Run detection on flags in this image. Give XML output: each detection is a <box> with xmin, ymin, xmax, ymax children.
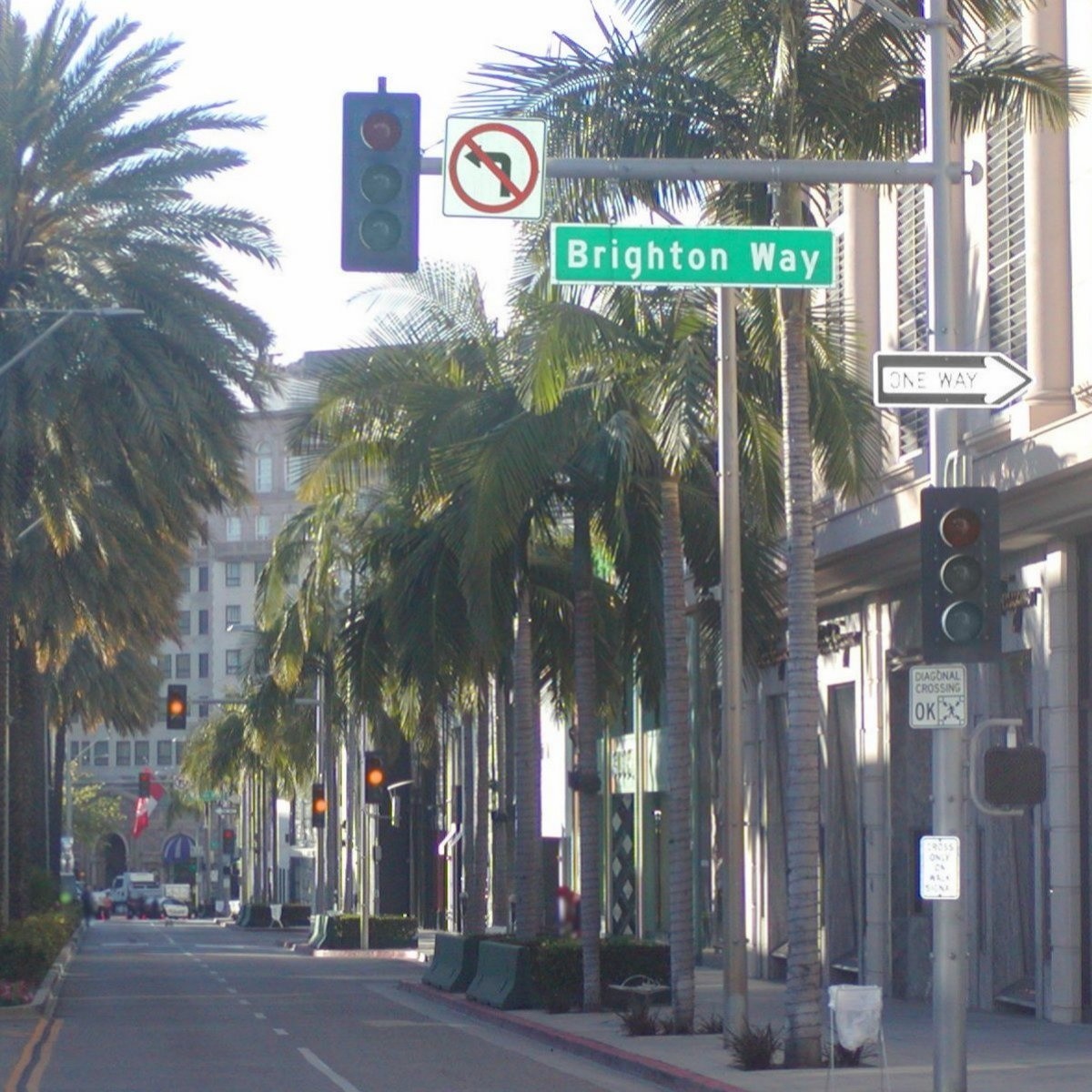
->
<box><xmin>131</xmin><ymin>764</ymin><xmax>166</xmax><ymax>838</ymax></box>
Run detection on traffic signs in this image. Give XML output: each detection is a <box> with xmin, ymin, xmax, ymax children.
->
<box><xmin>873</xmin><ymin>346</ymin><xmax>1033</xmax><ymax>415</ymax></box>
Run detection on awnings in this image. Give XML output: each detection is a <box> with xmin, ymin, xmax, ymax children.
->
<box><xmin>162</xmin><ymin>834</ymin><xmax>197</xmax><ymax>858</ymax></box>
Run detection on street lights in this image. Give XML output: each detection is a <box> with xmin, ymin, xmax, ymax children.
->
<box><xmin>224</xmin><ymin>623</ymin><xmax>339</xmax><ymax>923</ymax></box>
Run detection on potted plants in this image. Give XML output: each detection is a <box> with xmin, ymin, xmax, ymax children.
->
<box><xmin>236</xmin><ymin>901</ymin><xmax>673</xmax><ymax>1010</ymax></box>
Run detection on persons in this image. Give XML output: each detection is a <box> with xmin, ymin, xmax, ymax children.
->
<box><xmin>554</xmin><ymin>885</ymin><xmax>602</xmax><ymax>941</ymax></box>
<box><xmin>81</xmin><ymin>883</ymin><xmax>114</xmax><ymax>928</ymax></box>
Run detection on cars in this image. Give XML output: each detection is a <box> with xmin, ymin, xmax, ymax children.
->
<box><xmin>89</xmin><ymin>872</ymin><xmax>198</xmax><ymax>920</ymax></box>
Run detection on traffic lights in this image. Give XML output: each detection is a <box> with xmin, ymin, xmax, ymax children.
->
<box><xmin>166</xmin><ymin>686</ymin><xmax>188</xmax><ymax>731</ymax></box>
<box><xmin>979</xmin><ymin>744</ymin><xmax>1051</xmax><ymax>811</ymax></box>
<box><xmin>223</xmin><ymin>824</ymin><xmax>234</xmax><ymax>855</ymax></box>
<box><xmin>139</xmin><ymin>773</ymin><xmax>149</xmax><ymax>798</ymax></box>
<box><xmin>342</xmin><ymin>85</ymin><xmax>419</xmax><ymax>279</ymax></box>
<box><xmin>359</xmin><ymin>751</ymin><xmax>389</xmax><ymax>805</ymax></box>
<box><xmin>917</xmin><ymin>479</ymin><xmax>1006</xmax><ymax>670</ymax></box>
<box><xmin>311</xmin><ymin>782</ymin><xmax>325</xmax><ymax>828</ymax></box>
